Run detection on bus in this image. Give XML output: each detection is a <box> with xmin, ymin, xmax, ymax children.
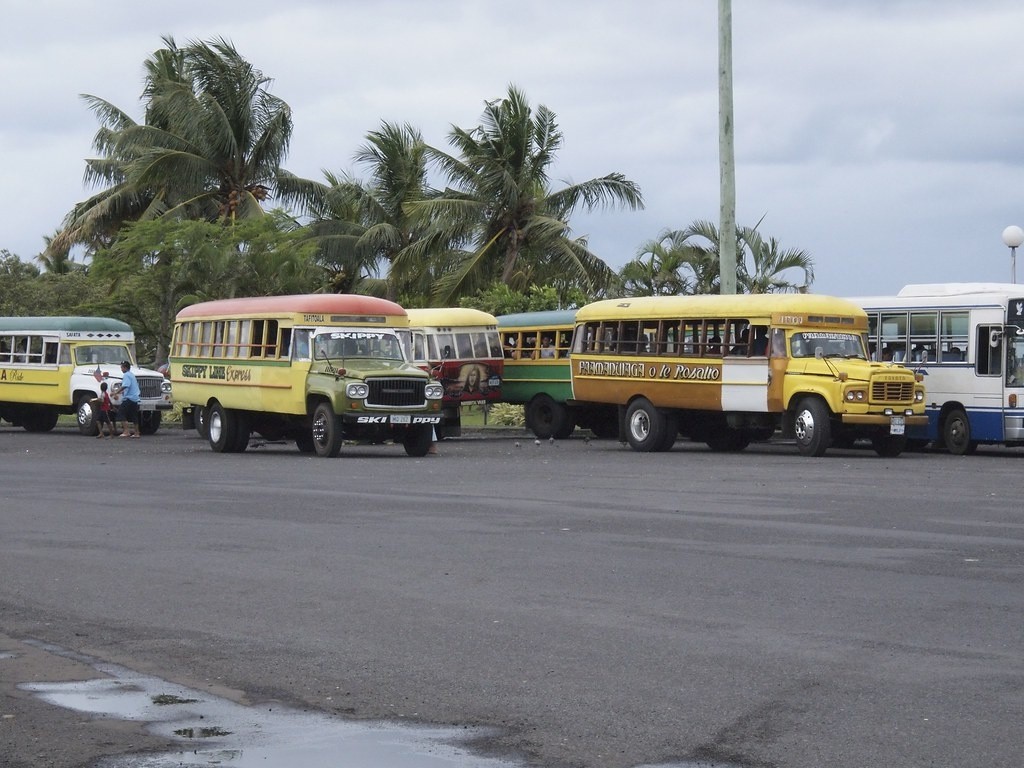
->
<box><xmin>569</xmin><ymin>293</ymin><xmax>929</xmax><ymax>459</ymax></box>
<box><xmin>494</xmin><ymin>308</ymin><xmax>620</xmax><ymax>440</ymax></box>
<box><xmin>0</xmin><ymin>316</ymin><xmax>173</xmax><ymax>435</ymax></box>
<box><xmin>402</xmin><ymin>307</ymin><xmax>504</xmax><ymax>440</ymax></box>
<box><xmin>841</xmin><ymin>280</ymin><xmax>1023</xmax><ymax>457</ymax></box>
<box><xmin>166</xmin><ymin>294</ymin><xmax>448</xmax><ymax>458</ymax></box>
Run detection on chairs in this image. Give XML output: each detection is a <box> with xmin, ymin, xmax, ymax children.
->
<box><xmin>102</xmin><ymin>348</ymin><xmax>117</xmax><ymax>361</ymax></box>
<box><xmin>927</xmin><ymin>355</ymin><xmax>936</xmax><ymax>361</ymax></box>
<box><xmin>332</xmin><ymin>339</ymin><xmax>358</xmax><ymax>355</ymax></box>
<box><xmin>942</xmin><ymin>354</ymin><xmax>960</xmax><ymax>361</ymax></box>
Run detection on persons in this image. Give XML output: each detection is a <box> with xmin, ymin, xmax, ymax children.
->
<box><xmin>707</xmin><ymin>336</ymin><xmax>721</xmax><ymax>354</ymax></box>
<box><xmin>332</xmin><ymin>338</ymin><xmax>358</xmax><ymax>356</ymax></box>
<box><xmin>870</xmin><ymin>341</ymin><xmax>961</xmax><ymax>362</ymax></box>
<box><xmin>110</xmin><ymin>361</ymin><xmax>141</xmax><ymax>438</ymax></box>
<box><xmin>731</xmin><ymin>326</ymin><xmax>769</xmax><ymax>356</ymax></box>
<box><xmin>610</xmin><ymin>327</ymin><xmax>648</xmax><ymax>351</ymax></box>
<box><xmin>96</xmin><ymin>382</ymin><xmax>113</xmax><ymax>439</ymax></box>
<box><xmin>1013</xmin><ymin>355</ymin><xmax>1024</xmax><ymax>386</ymax></box>
<box><xmin>504</xmin><ymin>333</ymin><xmax>567</xmax><ymax>359</ymax></box>
<box><xmin>806</xmin><ymin>338</ymin><xmax>834</xmax><ymax>355</ymax></box>
<box><xmin>0</xmin><ymin>338</ymin><xmax>34</xmax><ymax>362</ymax></box>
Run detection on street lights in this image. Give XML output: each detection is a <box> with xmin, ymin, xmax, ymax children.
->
<box><xmin>1001</xmin><ymin>223</ymin><xmax>1024</xmax><ymax>282</ymax></box>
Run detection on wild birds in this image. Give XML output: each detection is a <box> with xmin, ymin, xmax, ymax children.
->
<box><xmin>535</xmin><ymin>435</ymin><xmax>541</xmax><ymax>446</ymax></box>
<box><xmin>515</xmin><ymin>442</ymin><xmax>519</xmax><ymax>448</ymax></box>
<box><xmin>549</xmin><ymin>435</ymin><xmax>555</xmax><ymax>445</ymax></box>
<box><xmin>584</xmin><ymin>435</ymin><xmax>594</xmax><ymax>446</ymax></box>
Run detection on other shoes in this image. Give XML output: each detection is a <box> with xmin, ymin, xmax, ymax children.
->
<box><xmin>130</xmin><ymin>435</ymin><xmax>140</xmax><ymax>437</ymax></box>
<box><xmin>105</xmin><ymin>436</ymin><xmax>113</xmax><ymax>440</ymax></box>
<box><xmin>120</xmin><ymin>433</ymin><xmax>131</xmax><ymax>437</ymax></box>
<box><xmin>97</xmin><ymin>433</ymin><xmax>104</xmax><ymax>438</ymax></box>
